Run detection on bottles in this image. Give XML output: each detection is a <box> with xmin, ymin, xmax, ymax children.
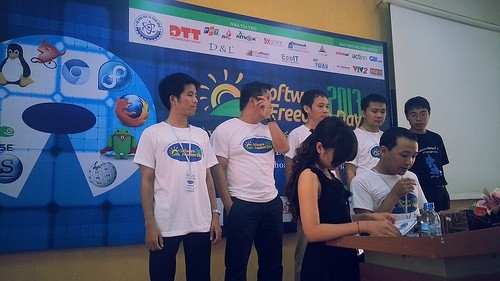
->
<box><xmin>420</xmin><ymin>203</ymin><xmax>442</xmax><ymax>237</ymax></box>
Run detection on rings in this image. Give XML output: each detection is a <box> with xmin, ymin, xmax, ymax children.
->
<box><xmin>357</xmin><ymin>221</ymin><xmax>362</xmax><ymax>234</ymax></box>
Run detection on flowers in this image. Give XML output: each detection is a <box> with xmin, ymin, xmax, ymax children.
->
<box><xmin>468</xmin><ymin>183</ymin><xmax>500</xmax><ymax>216</ymax></box>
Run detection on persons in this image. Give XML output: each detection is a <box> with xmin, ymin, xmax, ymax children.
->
<box><xmin>350</xmin><ymin>127</ymin><xmax>428</xmax><ymax>220</ymax></box>
<box><xmin>288</xmin><ymin>116</ymin><xmax>399</xmax><ymax>281</ymax></box>
<box><xmin>343</xmin><ymin>93</ymin><xmax>388</xmax><ymax>189</ymax></box>
<box><xmin>211</xmin><ymin>81</ymin><xmax>290</xmax><ymax>281</ymax></box>
<box><xmin>132</xmin><ymin>71</ymin><xmax>223</xmax><ymax>281</ymax></box>
<box><xmin>405</xmin><ymin>95</ymin><xmax>451</xmax><ymax>213</ymax></box>
<box><xmin>283</xmin><ymin>88</ymin><xmax>345</xmax><ymax>281</ymax></box>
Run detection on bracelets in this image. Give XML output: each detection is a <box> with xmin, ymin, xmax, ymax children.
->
<box><xmin>213</xmin><ymin>208</ymin><xmax>223</xmax><ymax>218</ymax></box>
<box><xmin>265</xmin><ymin>116</ymin><xmax>276</xmax><ymax>123</ymax></box>
